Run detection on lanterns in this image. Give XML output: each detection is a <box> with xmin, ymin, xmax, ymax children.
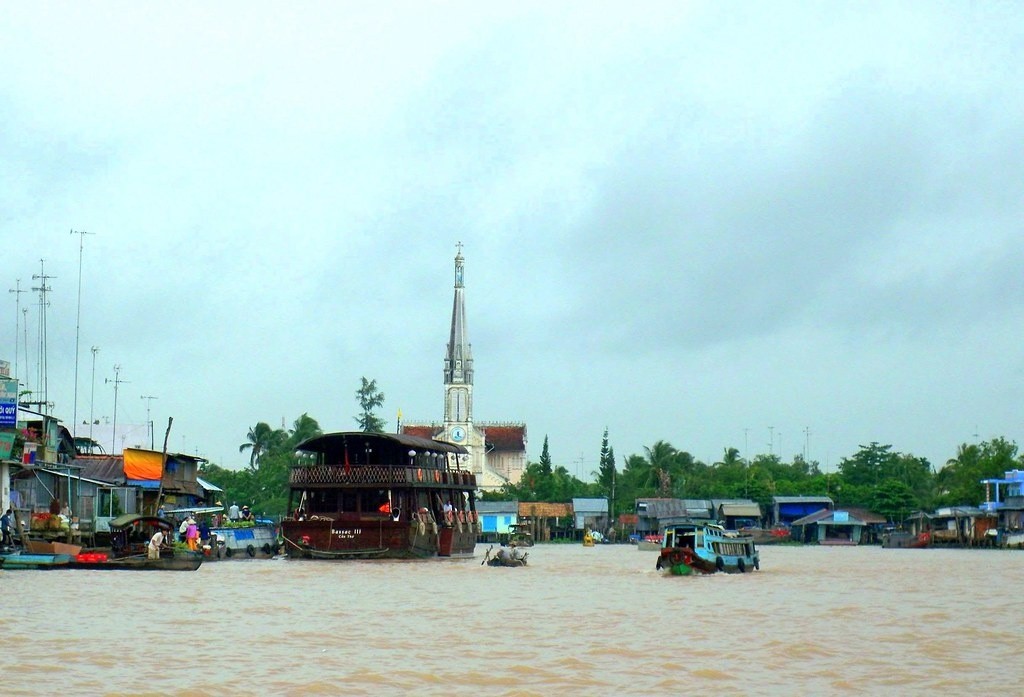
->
<box><xmin>409</xmin><ymin>450</ymin><xmax>469</xmax><ymax>464</ymax></box>
<box><xmin>295</xmin><ymin>449</ymin><xmax>315</xmax><ymax>465</ymax></box>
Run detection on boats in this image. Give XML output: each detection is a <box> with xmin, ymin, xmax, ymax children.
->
<box><xmin>282</xmin><ymin>430</ymin><xmax>481</xmax><ymax>561</ymax></box>
<box><xmin>656</xmin><ymin>523</ymin><xmax>760</xmax><ymax>575</ymax></box>
<box><xmin>636</xmin><ymin>534</ymin><xmax>664</xmax><ymax>551</ymax></box>
<box><xmin>583</xmin><ymin>533</ymin><xmax>594</xmax><ymax>547</ymax></box>
<box><xmin>487</xmin><ymin>552</ymin><xmax>528</xmax><ymax>566</ymax></box>
<box><xmin>0</xmin><ymin>512</ymin><xmax>206</xmax><ymax>571</ymax></box>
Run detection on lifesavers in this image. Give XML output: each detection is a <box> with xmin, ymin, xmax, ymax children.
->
<box><xmin>225</xmin><ymin>547</ymin><xmax>232</xmax><ymax>559</ymax></box>
<box><xmin>247</xmin><ymin>544</ymin><xmax>256</xmax><ymax>557</ymax></box>
<box><xmin>273</xmin><ymin>544</ymin><xmax>279</xmax><ymax>555</ymax></box>
<box><xmin>716</xmin><ymin>555</ymin><xmax>726</xmax><ymax>570</ymax></box>
<box><xmin>669</xmin><ymin>550</ymin><xmax>686</xmax><ymax>566</ymax></box>
<box><xmin>754</xmin><ymin>558</ymin><xmax>759</xmax><ymax>570</ymax></box>
<box><xmin>738</xmin><ymin>557</ymin><xmax>746</xmax><ymax>572</ymax></box>
<box><xmin>435</xmin><ymin>469</ymin><xmax>440</xmax><ymax>483</ymax></box>
<box><xmin>417</xmin><ymin>468</ymin><xmax>424</xmax><ymax>481</ymax></box>
<box><xmin>459</xmin><ymin>510</ymin><xmax>464</xmax><ymax>523</ymax></box>
<box><xmin>264</xmin><ymin>543</ymin><xmax>272</xmax><ymax>555</ymax></box>
<box><xmin>448</xmin><ymin>511</ymin><xmax>453</xmax><ymax>521</ymax></box>
<box><xmin>467</xmin><ymin>511</ymin><xmax>474</xmax><ymax>522</ymax></box>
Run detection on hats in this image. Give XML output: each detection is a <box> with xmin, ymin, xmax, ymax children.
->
<box><xmin>212</xmin><ymin>514</ymin><xmax>216</xmax><ymax>517</ymax></box>
<box><xmin>222</xmin><ymin>514</ymin><xmax>227</xmax><ymax>519</ymax></box>
<box><xmin>242</xmin><ymin>506</ymin><xmax>249</xmax><ymax>509</ymax></box>
<box><xmin>188</xmin><ymin>519</ymin><xmax>196</xmax><ymax>524</ymax></box>
<box><xmin>159</xmin><ymin>504</ymin><xmax>165</xmax><ymax>508</ymax></box>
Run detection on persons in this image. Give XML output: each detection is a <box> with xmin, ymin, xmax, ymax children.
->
<box><xmin>148</xmin><ymin>527</ymin><xmax>175</xmax><ymax>558</ymax></box>
<box><xmin>497</xmin><ymin>541</ymin><xmax>521</xmax><ymax>560</ymax></box>
<box><xmin>50</xmin><ymin>499</ymin><xmax>72</xmax><ymax>519</ymax></box>
<box><xmin>444</xmin><ymin>500</ymin><xmax>452</xmax><ymax>522</ymax></box>
<box><xmin>0</xmin><ymin>508</ymin><xmax>12</xmax><ymax>543</ymax></box>
<box><xmin>178</xmin><ymin>516</ymin><xmax>211</xmax><ymax>551</ymax></box>
<box><xmin>675</xmin><ymin>537</ymin><xmax>679</xmax><ymax>548</ymax></box>
<box><xmin>158</xmin><ymin>501</ymin><xmax>254</xmax><ymax>529</ymax></box>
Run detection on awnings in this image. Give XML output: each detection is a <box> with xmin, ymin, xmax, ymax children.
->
<box><xmin>197</xmin><ymin>477</ymin><xmax>225</xmax><ymax>492</ymax></box>
<box><xmin>473</xmin><ymin>514</ymin><xmax>517</xmax><ymax>534</ymax></box>
